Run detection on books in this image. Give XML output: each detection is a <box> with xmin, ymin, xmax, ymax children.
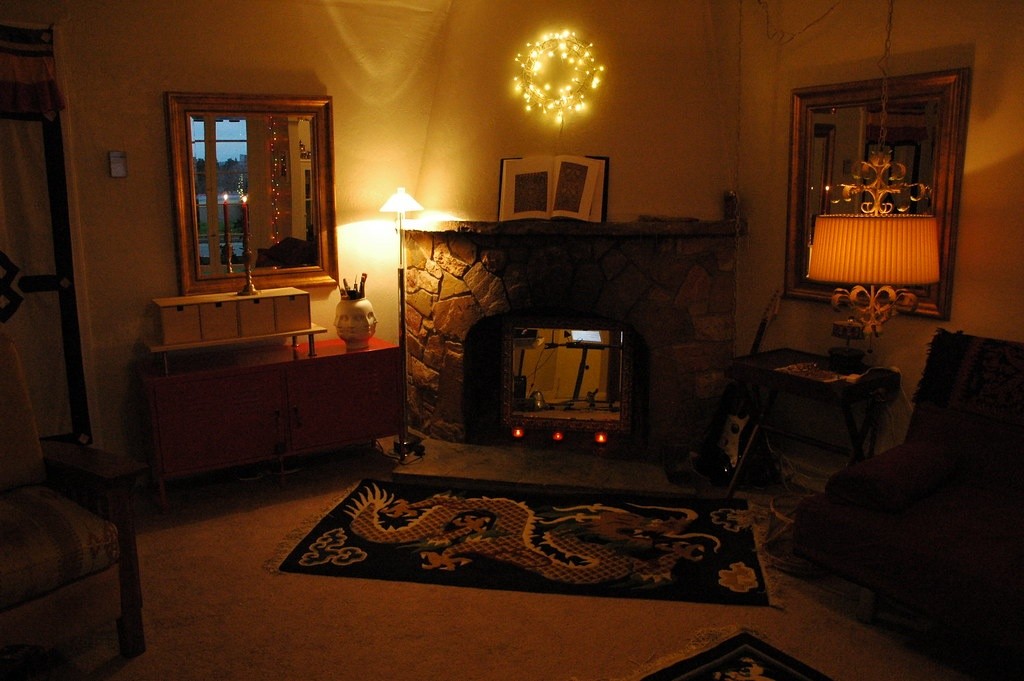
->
<box><xmin>496</xmin><ymin>154</ymin><xmax>611</xmax><ymax>226</ymax></box>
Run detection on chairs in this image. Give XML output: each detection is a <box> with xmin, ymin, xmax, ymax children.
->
<box><xmin>0</xmin><ymin>334</ymin><xmax>147</xmax><ymax>658</ymax></box>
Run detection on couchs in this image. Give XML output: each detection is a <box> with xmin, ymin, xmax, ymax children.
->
<box><xmin>793</xmin><ymin>328</ymin><xmax>1024</xmax><ymax>681</ymax></box>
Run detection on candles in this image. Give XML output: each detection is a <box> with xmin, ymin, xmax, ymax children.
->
<box><xmin>242</xmin><ymin>204</ymin><xmax>248</xmax><ymax>235</ymax></box>
<box><xmin>223</xmin><ymin>202</ymin><xmax>231</xmax><ymax>233</ymax></box>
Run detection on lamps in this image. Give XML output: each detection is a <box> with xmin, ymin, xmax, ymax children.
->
<box><xmin>808</xmin><ymin>0</ymin><xmax>944</xmax><ymax>337</ymax></box>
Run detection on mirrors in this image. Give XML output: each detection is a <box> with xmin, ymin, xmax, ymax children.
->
<box><xmin>782</xmin><ymin>66</ymin><xmax>971</xmax><ymax>320</ymax></box>
<box><xmin>166</xmin><ymin>91</ymin><xmax>340</xmax><ymax>296</ymax></box>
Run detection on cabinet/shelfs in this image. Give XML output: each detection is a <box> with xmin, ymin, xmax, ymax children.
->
<box><xmin>141</xmin><ymin>336</ymin><xmax>406</xmax><ymax>506</ymax></box>
<box><xmin>144</xmin><ymin>286</ymin><xmax>327</xmax><ymax>374</ymax></box>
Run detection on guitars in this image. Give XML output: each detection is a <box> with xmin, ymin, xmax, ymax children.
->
<box><xmin>696</xmin><ymin>283</ymin><xmax>786</xmax><ymax>487</ymax></box>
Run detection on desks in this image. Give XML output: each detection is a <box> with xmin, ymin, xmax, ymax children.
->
<box><xmin>730</xmin><ymin>347</ymin><xmax>900</xmax><ymax>466</ymax></box>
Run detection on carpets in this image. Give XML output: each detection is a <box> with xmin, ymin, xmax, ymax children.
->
<box><xmin>264</xmin><ymin>477</ymin><xmax>786</xmax><ymax>610</ymax></box>
<box><xmin>616</xmin><ymin>624</ymin><xmax>837</xmax><ymax>681</ymax></box>
